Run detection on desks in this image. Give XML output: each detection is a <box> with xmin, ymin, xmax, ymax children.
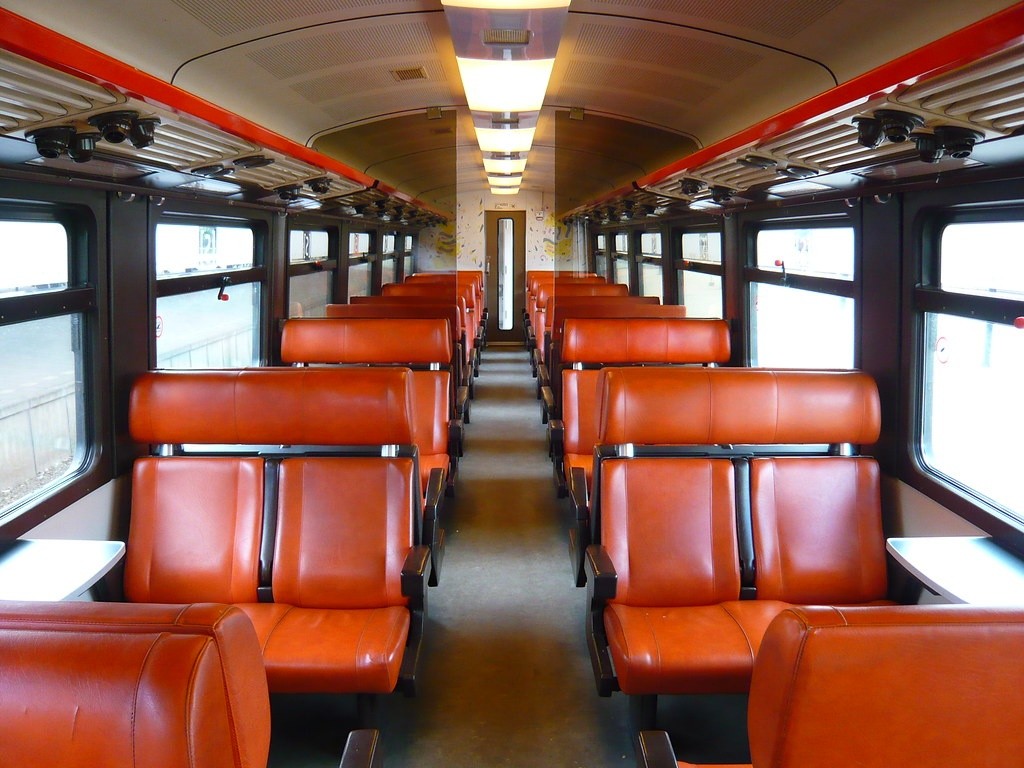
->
<box><xmin>0</xmin><ymin>538</ymin><xmax>127</xmax><ymax>604</ymax></box>
<box><xmin>886</xmin><ymin>534</ymin><xmax>1024</xmax><ymax>609</ymax></box>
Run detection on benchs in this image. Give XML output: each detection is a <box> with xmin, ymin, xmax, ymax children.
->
<box><xmin>524</xmin><ymin>267</ymin><xmax>1024</xmax><ymax>768</ymax></box>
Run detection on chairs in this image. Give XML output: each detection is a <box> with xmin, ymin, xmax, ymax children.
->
<box><xmin>0</xmin><ymin>270</ymin><xmax>488</xmax><ymax>767</ymax></box>
